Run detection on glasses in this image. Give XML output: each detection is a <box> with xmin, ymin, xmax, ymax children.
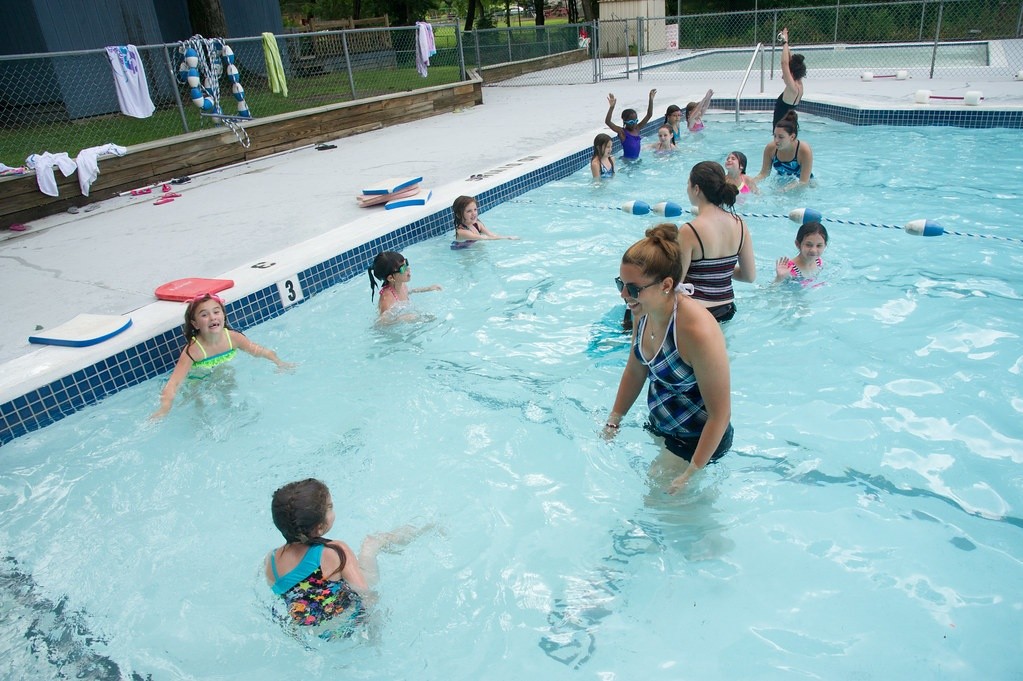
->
<box><xmin>624</xmin><ymin>119</ymin><xmax>638</xmax><ymax>125</ymax></box>
<box><xmin>392</xmin><ymin>258</ymin><xmax>409</xmax><ymax>275</ymax></box>
<box><xmin>614</xmin><ymin>275</ymin><xmax>663</xmax><ymax>298</ymax></box>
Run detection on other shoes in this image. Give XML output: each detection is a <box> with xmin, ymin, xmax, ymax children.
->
<box><xmin>83</xmin><ymin>201</ymin><xmax>101</xmax><ymax>212</ymax></box>
<box><xmin>132</xmin><ymin>188</ymin><xmax>151</xmax><ymax>195</ymax></box>
<box><xmin>67</xmin><ymin>206</ymin><xmax>79</xmax><ymax>215</ymax></box>
<box><xmin>162</xmin><ymin>184</ymin><xmax>170</xmax><ymax>192</ymax></box>
<box><xmin>171</xmin><ymin>176</ymin><xmax>192</xmax><ymax>185</ymax></box>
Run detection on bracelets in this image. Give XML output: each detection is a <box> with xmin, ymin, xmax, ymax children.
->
<box><xmin>606</xmin><ymin>422</ymin><xmax>621</xmax><ymax>429</ymax></box>
<box><xmin>782</xmin><ymin>40</ymin><xmax>788</xmax><ymax>45</ymax></box>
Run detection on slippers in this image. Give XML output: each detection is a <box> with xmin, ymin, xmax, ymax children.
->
<box><xmin>161</xmin><ymin>191</ymin><xmax>182</xmax><ymax>197</ymax></box>
<box><xmin>9</xmin><ymin>223</ymin><xmax>26</xmax><ymax>231</ymax></box>
<box><xmin>153</xmin><ymin>197</ymin><xmax>174</xmax><ymax>206</ymax></box>
<box><xmin>314</xmin><ymin>143</ymin><xmax>336</xmax><ymax>149</ymax></box>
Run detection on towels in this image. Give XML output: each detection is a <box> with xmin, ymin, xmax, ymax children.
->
<box><xmin>25</xmin><ymin>151</ymin><xmax>78</xmax><ymax>197</ymax></box>
<box><xmin>105</xmin><ymin>45</ymin><xmax>155</xmax><ymax>120</ymax></box>
<box><xmin>415</xmin><ymin>21</ymin><xmax>437</xmax><ymax>78</ymax></box>
<box><xmin>76</xmin><ymin>142</ymin><xmax>127</xmax><ymax>197</ymax></box>
<box><xmin>261</xmin><ymin>32</ymin><xmax>288</xmax><ymax>98</ymax></box>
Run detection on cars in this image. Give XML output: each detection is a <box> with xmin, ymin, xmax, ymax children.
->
<box><xmin>504</xmin><ymin>7</ymin><xmax>524</xmax><ymax>15</ymax></box>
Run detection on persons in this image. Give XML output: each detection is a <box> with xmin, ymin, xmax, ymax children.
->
<box><xmin>641</xmin><ymin>123</ymin><xmax>677</xmax><ymax>151</ymax></box>
<box><xmin>367</xmin><ymin>251</ymin><xmax>443</xmax><ymax>328</ymax></box>
<box><xmin>677</xmin><ymin>161</ymin><xmax>757</xmax><ymax>324</ymax></box>
<box><xmin>772</xmin><ymin>222</ymin><xmax>829</xmax><ymax>290</ymax></box>
<box><xmin>686</xmin><ymin>89</ymin><xmax>714</xmax><ymax>132</ymax></box>
<box><xmin>725</xmin><ymin>151</ymin><xmax>760</xmax><ymax>195</ymax></box>
<box><xmin>604</xmin><ymin>88</ymin><xmax>657</xmax><ymax>161</ymax></box>
<box><xmin>753</xmin><ymin>110</ymin><xmax>817</xmax><ymax>195</ymax></box>
<box><xmin>152</xmin><ymin>292</ymin><xmax>298</xmax><ymax>422</ymax></box>
<box><xmin>773</xmin><ymin>27</ymin><xmax>807</xmax><ymax>134</ymax></box>
<box><xmin>590</xmin><ymin>133</ymin><xmax>616</xmax><ymax>182</ymax></box>
<box><xmin>664</xmin><ymin>104</ymin><xmax>681</xmax><ymax>142</ymax></box>
<box><xmin>599</xmin><ymin>223</ymin><xmax>734</xmax><ymax>498</ymax></box>
<box><xmin>265</xmin><ymin>478</ymin><xmax>437</xmax><ymax>658</ymax></box>
<box><xmin>451</xmin><ymin>196</ymin><xmax>521</xmax><ymax>250</ymax></box>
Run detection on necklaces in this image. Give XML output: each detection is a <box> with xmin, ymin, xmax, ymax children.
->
<box><xmin>648</xmin><ymin>314</ymin><xmax>672</xmax><ymax>340</ymax></box>
